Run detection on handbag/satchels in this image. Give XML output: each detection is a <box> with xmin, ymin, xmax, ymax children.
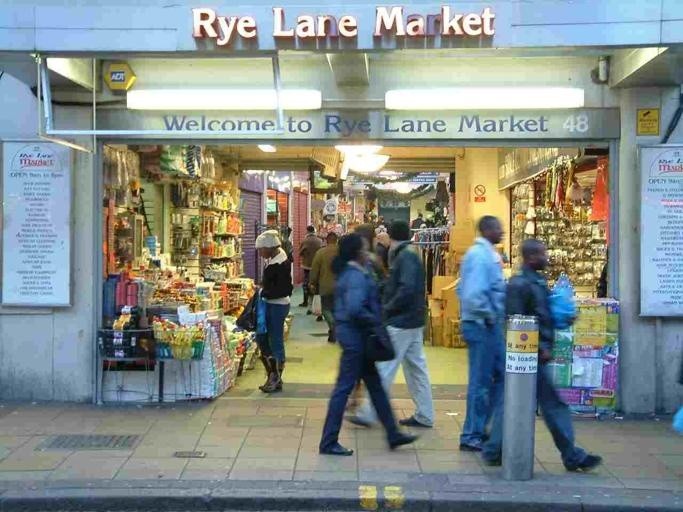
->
<box><xmin>236</xmin><ymin>292</ymin><xmax>257</xmax><ymax>331</ymax></box>
<box><xmin>358</xmin><ymin>325</ymin><xmax>395</xmax><ymax>362</ymax></box>
<box><xmin>307</xmin><ymin>294</ymin><xmax>322</xmax><ymax>316</ymax></box>
<box><xmin>256</xmin><ymin>299</ymin><xmax>268</xmax><ymax>335</ymax></box>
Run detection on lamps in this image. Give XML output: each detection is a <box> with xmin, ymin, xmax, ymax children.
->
<box><xmin>125</xmin><ymin>87</ymin><xmax>322</xmax><ymax>113</ymax></box>
<box><xmin>384</xmin><ymin>86</ymin><xmax>585</xmax><ymax>110</ymax></box>
<box><xmin>335</xmin><ymin>144</ymin><xmax>392</xmax><ymax>174</ymax></box>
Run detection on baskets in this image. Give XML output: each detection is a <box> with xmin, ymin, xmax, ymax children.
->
<box><xmin>97</xmin><ymin>330</ymin><xmax>153</xmax><ymax>358</ymax></box>
<box><xmin>154</xmin><ymin>329</ymin><xmax>207</xmax><ymax>360</ymax></box>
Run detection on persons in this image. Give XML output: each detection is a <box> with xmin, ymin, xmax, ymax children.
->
<box><xmin>319</xmin><ymin>233</ymin><xmax>420</xmax><ymax>455</ymax></box>
<box><xmin>411</xmin><ymin>214</ymin><xmax>425</xmax><ymax>229</ymax></box>
<box><xmin>456</xmin><ymin>216</ymin><xmax>506</xmax><ymax>452</ymax></box>
<box><xmin>344</xmin><ymin>221</ymin><xmax>434</xmax><ymax>427</ymax></box>
<box><xmin>252</xmin><ymin>229</ymin><xmax>293</xmax><ymax>394</ymax></box>
<box><xmin>280</xmin><ymin>225</ymin><xmax>390</xmax><ymax>343</ymax></box>
<box><xmin>482</xmin><ymin>239</ymin><xmax>603</xmax><ymax>472</ymax></box>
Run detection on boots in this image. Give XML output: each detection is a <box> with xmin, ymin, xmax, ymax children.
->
<box><xmin>259</xmin><ymin>353</ymin><xmax>285</xmax><ymax>393</ymax></box>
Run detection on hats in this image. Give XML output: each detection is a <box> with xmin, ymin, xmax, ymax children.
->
<box><xmin>255</xmin><ymin>230</ymin><xmax>281</xmax><ymax>248</ymax></box>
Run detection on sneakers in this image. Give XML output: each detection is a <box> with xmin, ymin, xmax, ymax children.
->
<box><xmin>388</xmin><ymin>432</ymin><xmax>415</xmax><ymax>447</ymax></box>
<box><xmin>399</xmin><ymin>414</ymin><xmax>433</xmax><ymax>427</ymax></box>
<box><xmin>320</xmin><ymin>443</ymin><xmax>353</xmax><ymax>455</ymax></box>
<box><xmin>566</xmin><ymin>454</ymin><xmax>601</xmax><ymax>471</ymax></box>
<box><xmin>329</xmin><ymin>336</ymin><xmax>336</xmax><ymax>342</ymax></box>
<box><xmin>481</xmin><ymin>449</ymin><xmax>502</xmax><ymax>465</ymax></box>
<box><xmin>459</xmin><ymin>435</ymin><xmax>487</xmax><ymax>452</ymax></box>
<box><xmin>344</xmin><ymin>415</ymin><xmax>371</xmax><ymax>427</ymax></box>
<box><xmin>299</xmin><ymin>303</ymin><xmax>307</xmax><ymax>306</ymax></box>
